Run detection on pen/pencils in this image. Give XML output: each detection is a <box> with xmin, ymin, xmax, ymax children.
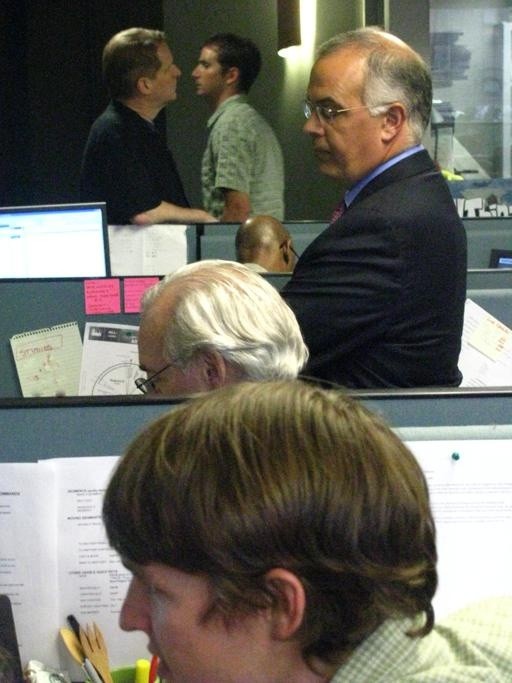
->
<box><xmin>68</xmin><ymin>615</ymin><xmax>158</xmax><ymax>683</ymax></box>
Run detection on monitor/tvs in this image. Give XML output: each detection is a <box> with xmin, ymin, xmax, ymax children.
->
<box><xmin>488</xmin><ymin>249</ymin><xmax>512</xmax><ymax>268</ymax></box>
<box><xmin>0</xmin><ymin>202</ymin><xmax>111</xmax><ymax>278</ymax></box>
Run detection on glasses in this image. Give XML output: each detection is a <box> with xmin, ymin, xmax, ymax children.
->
<box><xmin>134</xmin><ymin>355</ymin><xmax>183</xmax><ymax>395</ymax></box>
<box><xmin>304</xmin><ymin>100</ymin><xmax>396</xmax><ymax>126</ymax></box>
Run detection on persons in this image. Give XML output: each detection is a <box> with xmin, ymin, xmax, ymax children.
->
<box><xmin>234</xmin><ymin>215</ymin><xmax>295</xmax><ymax>273</ymax></box>
<box><xmin>280</xmin><ymin>27</ymin><xmax>466</xmax><ymax>390</ymax></box>
<box><xmin>192</xmin><ymin>31</ymin><xmax>285</xmax><ymax>223</ymax></box>
<box><xmin>102</xmin><ymin>380</ymin><xmax>512</xmax><ymax>683</ymax></box>
<box><xmin>80</xmin><ymin>27</ymin><xmax>221</xmax><ymax>224</ymax></box>
<box><xmin>137</xmin><ymin>258</ymin><xmax>310</xmax><ymax>395</ymax></box>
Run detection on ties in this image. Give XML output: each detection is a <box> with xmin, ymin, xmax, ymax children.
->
<box><xmin>329</xmin><ymin>201</ymin><xmax>347</xmax><ymax>224</ymax></box>
